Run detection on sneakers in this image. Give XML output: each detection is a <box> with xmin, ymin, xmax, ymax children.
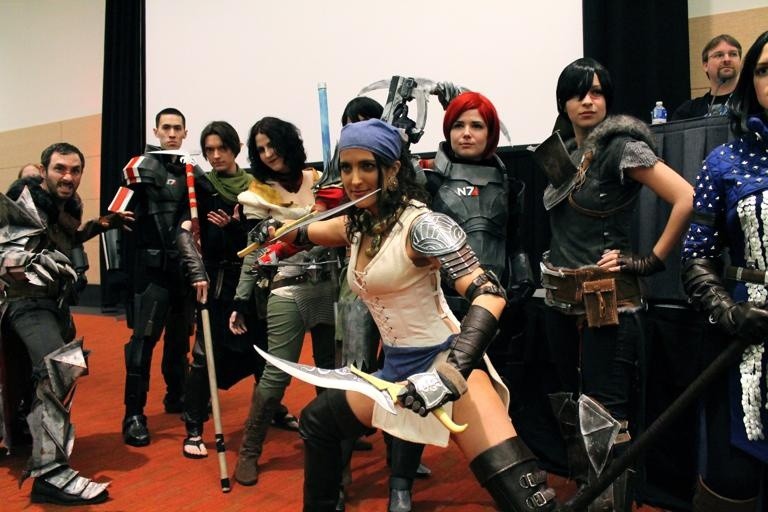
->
<box><xmin>28</xmin><ymin>465</ymin><xmax>110</xmax><ymax>505</ymax></box>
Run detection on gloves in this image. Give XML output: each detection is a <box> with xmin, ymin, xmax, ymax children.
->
<box><xmin>397</xmin><ymin>359</ymin><xmax>469</xmax><ymax>420</ymax></box>
<box><xmin>246</xmin><ymin>215</ymin><xmax>285</xmax><ymax>245</ymax></box>
<box><xmin>616</xmin><ymin>246</ymin><xmax>668</xmax><ymax>277</ymax></box>
<box><xmin>680</xmin><ymin>256</ymin><xmax>768</xmax><ymax>342</ymax></box>
<box><xmin>505</xmin><ymin>281</ymin><xmax>535</xmax><ymax>314</ymax></box>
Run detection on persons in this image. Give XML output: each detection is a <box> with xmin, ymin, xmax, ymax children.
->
<box><xmin>105</xmin><ymin>57</ymin><xmax>696</xmax><ymax>511</ymax></box>
<box><xmin>17</xmin><ymin>161</ymin><xmax>41</xmax><ymax>178</ymax></box>
<box><xmin>681</xmin><ymin>29</ymin><xmax>767</xmax><ymax>511</ymax></box>
<box><xmin>1</xmin><ymin>140</ymin><xmax>134</xmax><ymax>504</ymax></box>
<box><xmin>670</xmin><ymin>33</ymin><xmax>742</xmax><ymax>120</ymax></box>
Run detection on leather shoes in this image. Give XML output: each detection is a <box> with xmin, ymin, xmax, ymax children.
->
<box><xmin>414</xmin><ymin>461</ymin><xmax>433</xmax><ymax>478</ymax></box>
<box><xmin>163</xmin><ymin>389</ymin><xmax>214</xmax><ymax>415</ymax></box>
<box><xmin>389</xmin><ymin>486</ymin><xmax>413</xmax><ymax>512</ymax></box>
<box><xmin>121</xmin><ymin>413</ymin><xmax>153</xmax><ymax>447</ymax></box>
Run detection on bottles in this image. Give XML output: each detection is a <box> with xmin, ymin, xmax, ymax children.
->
<box><xmin>652</xmin><ymin>102</ymin><xmax>669</xmax><ymax>125</ymax></box>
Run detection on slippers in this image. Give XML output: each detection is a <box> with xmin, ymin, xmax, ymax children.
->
<box><xmin>183</xmin><ymin>432</ymin><xmax>209</xmax><ymax>462</ymax></box>
<box><xmin>269</xmin><ymin>414</ymin><xmax>303</xmax><ymax>432</ymax></box>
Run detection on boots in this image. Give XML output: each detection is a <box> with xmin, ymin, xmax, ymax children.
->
<box><xmin>578</xmin><ymin>393</ymin><xmax>632</xmax><ymax>512</ymax></box>
<box><xmin>234</xmin><ymin>381</ymin><xmax>278</xmax><ymax>486</ymax></box>
<box><xmin>548</xmin><ymin>390</ymin><xmax>593</xmax><ymax>512</ymax></box>
<box><xmin>295</xmin><ymin>388</ymin><xmax>377</xmax><ymax>511</ymax></box>
<box><xmin>468</xmin><ymin>436</ymin><xmax>562</xmax><ymax>512</ymax></box>
